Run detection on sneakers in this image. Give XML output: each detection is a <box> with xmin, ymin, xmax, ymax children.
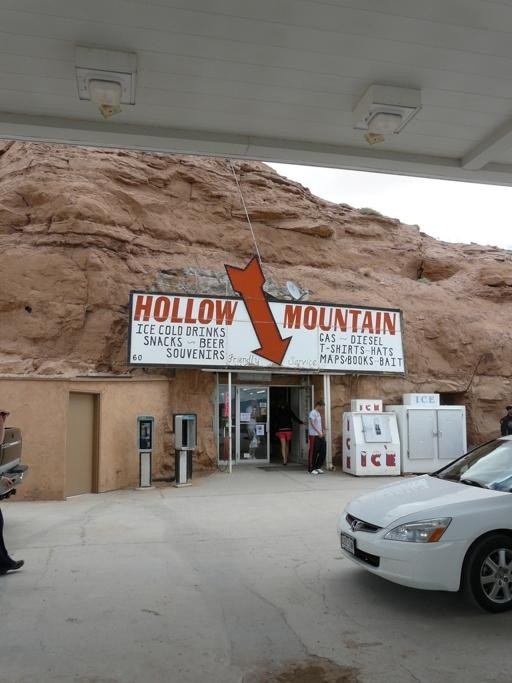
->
<box><xmin>310</xmin><ymin>469</ymin><xmax>324</xmax><ymax>474</ymax></box>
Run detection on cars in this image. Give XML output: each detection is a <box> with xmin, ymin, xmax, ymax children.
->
<box><xmin>335</xmin><ymin>433</ymin><xmax>512</xmax><ymax>615</ymax></box>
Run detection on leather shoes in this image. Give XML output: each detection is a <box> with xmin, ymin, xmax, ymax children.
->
<box><xmin>0</xmin><ymin>559</ymin><xmax>24</xmax><ymax>574</ymax></box>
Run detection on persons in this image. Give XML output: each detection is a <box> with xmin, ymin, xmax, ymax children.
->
<box><xmin>501</xmin><ymin>406</ymin><xmax>512</xmax><ymax>436</ymax></box>
<box><xmin>271</xmin><ymin>398</ymin><xmax>305</xmax><ymax>465</ymax></box>
<box><xmin>245</xmin><ymin>398</ymin><xmax>259</xmax><ymax>459</ymax></box>
<box><xmin>0</xmin><ymin>404</ymin><xmax>24</xmax><ymax>576</ymax></box>
<box><xmin>308</xmin><ymin>400</ymin><xmax>327</xmax><ymax>475</ymax></box>
